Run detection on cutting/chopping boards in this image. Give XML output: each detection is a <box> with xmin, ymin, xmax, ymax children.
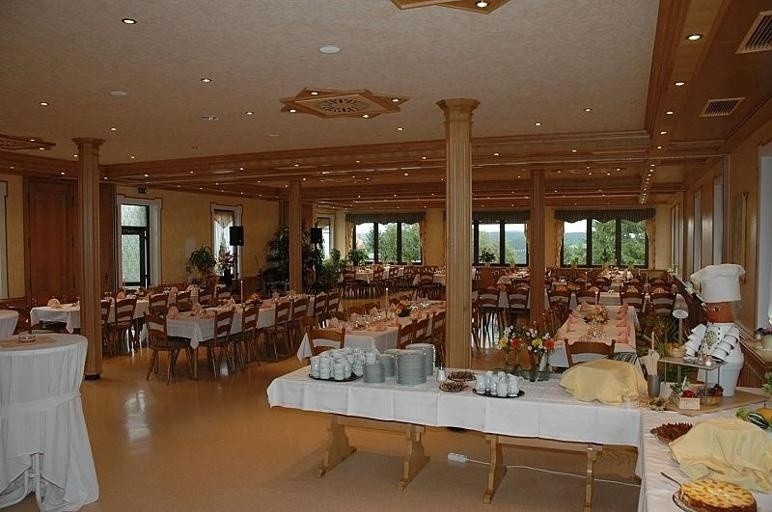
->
<box><xmin>673</xmin><ymin>491</ymin><xmax>693</xmax><ymax>512</ymax></box>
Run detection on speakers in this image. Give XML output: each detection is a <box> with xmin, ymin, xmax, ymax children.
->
<box><xmin>230</xmin><ymin>226</ymin><xmax>244</xmax><ymax>246</ymax></box>
<box><xmin>310</xmin><ymin>228</ymin><xmax>322</xmax><ymax>244</ymax></box>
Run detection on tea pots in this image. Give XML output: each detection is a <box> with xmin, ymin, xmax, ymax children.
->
<box><xmin>473</xmin><ymin>371</ymin><xmax>524</xmax><ymax>397</ymax></box>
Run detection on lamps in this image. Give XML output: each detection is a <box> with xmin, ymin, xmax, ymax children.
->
<box><xmin>310</xmin><ymin>228</ymin><xmax>321</xmax><ymax>251</ymax></box>
<box><xmin>229</xmin><ymin>225</ymin><xmax>244</xmax><ymax>294</ymax></box>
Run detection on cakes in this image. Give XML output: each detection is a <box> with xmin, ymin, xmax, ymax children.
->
<box><xmin>680</xmin><ymin>479</ymin><xmax>758</xmax><ymax>512</ymax></box>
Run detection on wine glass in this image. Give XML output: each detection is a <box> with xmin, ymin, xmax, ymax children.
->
<box><xmin>104</xmin><ymin>292</ymin><xmax>112</xmax><ymax>301</ymax></box>
<box><xmin>76</xmin><ymin>297</ymin><xmax>81</xmax><ymax>308</ymax></box>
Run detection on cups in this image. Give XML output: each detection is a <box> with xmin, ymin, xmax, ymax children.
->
<box><xmin>127</xmin><ymin>295</ymin><xmax>136</xmax><ymax>299</ymax></box>
<box><xmin>711</xmin><ymin>327</ymin><xmax>740</xmax><ymax>360</ymax></box>
<box><xmin>681</xmin><ymin>324</ymin><xmax>706</xmax><ymax>357</ymax></box>
<box><xmin>648</xmin><ymin>375</ymin><xmax>660</xmax><ymax>398</ymax></box>
<box><xmin>310</xmin><ymin>348</ymin><xmax>375</xmax><ymax>381</ymax></box>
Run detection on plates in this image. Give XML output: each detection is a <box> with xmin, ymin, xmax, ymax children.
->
<box><xmin>649</xmin><ymin>429</ymin><xmax>672</xmax><ymax>444</ymax></box>
<box><xmin>449</xmin><ymin>374</ymin><xmax>476</xmax><ymax>382</ymax></box>
<box><xmin>439</xmin><ymin>383</ymin><xmax>468</xmax><ymax>392</ymax></box>
<box><xmin>364</xmin><ymin>341</ymin><xmax>436</xmax><ymax>385</ymax></box>
<box><xmin>473</xmin><ymin>388</ymin><xmax>525</xmax><ymax>398</ymax></box>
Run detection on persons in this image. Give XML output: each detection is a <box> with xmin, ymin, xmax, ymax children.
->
<box><xmin>681</xmin><ymin>263</ymin><xmax>747</xmax><ymax>399</ymax></box>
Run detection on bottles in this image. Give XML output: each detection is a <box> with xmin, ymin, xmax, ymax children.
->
<box><xmin>438</xmin><ymin>362</ymin><xmax>447</xmax><ymax>382</ymax></box>
